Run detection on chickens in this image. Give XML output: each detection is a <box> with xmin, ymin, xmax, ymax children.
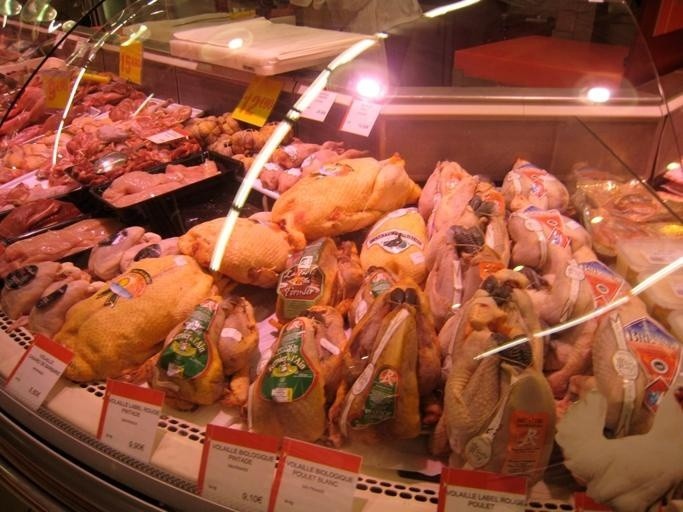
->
<box><xmin>554</xmin><ymin>384</ymin><xmax>682</xmax><ymax>508</ymax></box>
<box><xmin>3</xmin><ymin>152</ymin><xmax>683</xmax><ymax>487</ymax></box>
